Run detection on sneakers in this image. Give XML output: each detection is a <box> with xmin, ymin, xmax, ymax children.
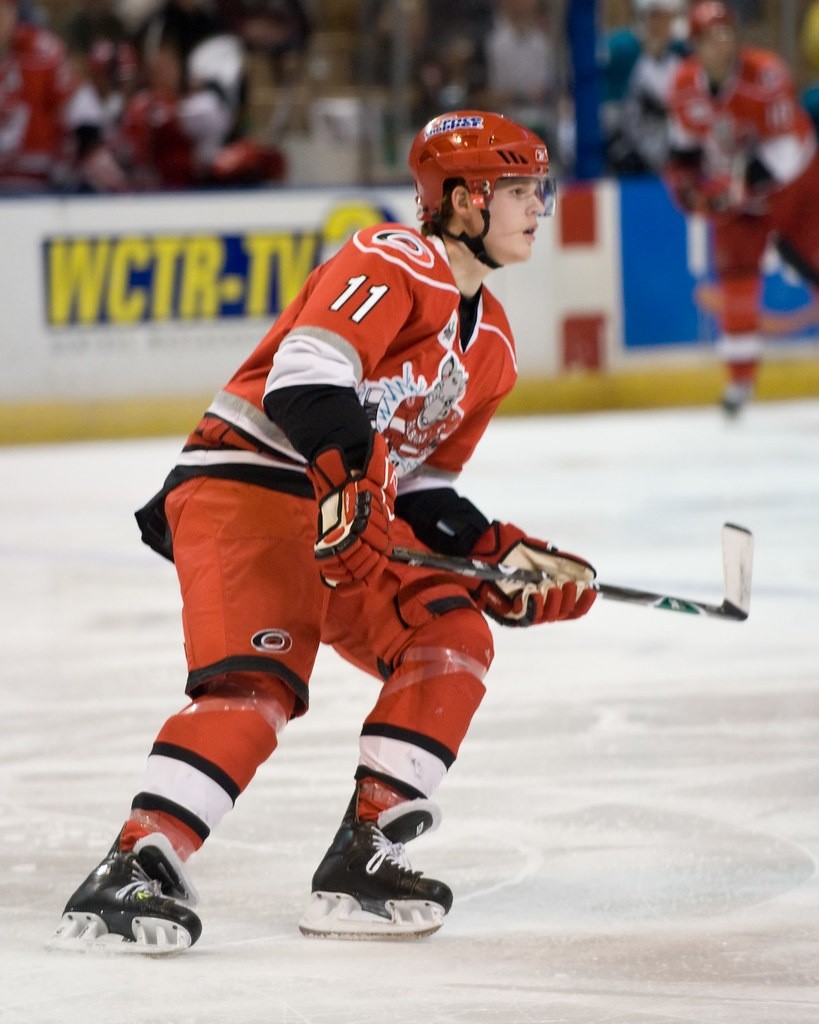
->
<box><xmin>295</xmin><ymin>785</ymin><xmax>452</xmax><ymax>936</ymax></box>
<box><xmin>44</xmin><ymin>822</ymin><xmax>202</xmax><ymax>955</ymax></box>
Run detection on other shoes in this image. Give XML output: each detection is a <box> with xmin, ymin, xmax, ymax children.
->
<box><xmin>714</xmin><ymin>361</ymin><xmax>755</xmax><ymax>414</ymax></box>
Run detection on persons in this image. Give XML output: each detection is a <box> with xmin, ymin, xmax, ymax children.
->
<box><xmin>34</xmin><ymin>108</ymin><xmax>600</xmax><ymax>951</ymax></box>
<box><xmin>664</xmin><ymin>0</ymin><xmax>819</xmax><ymax>411</ymax></box>
<box><xmin>0</xmin><ymin>0</ymin><xmax>697</xmax><ymax>197</ymax></box>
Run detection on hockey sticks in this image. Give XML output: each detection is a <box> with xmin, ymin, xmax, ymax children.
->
<box><xmin>390</xmin><ymin>522</ymin><xmax>754</xmax><ymax>621</ymax></box>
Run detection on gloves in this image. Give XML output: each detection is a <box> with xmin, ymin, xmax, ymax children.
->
<box><xmin>454</xmin><ymin>521</ymin><xmax>599</xmax><ymax>628</ymax></box>
<box><xmin>731</xmin><ymin>162</ymin><xmax>774</xmax><ymax>214</ymax></box>
<box><xmin>667</xmin><ymin>147</ymin><xmax>704</xmax><ymax>212</ymax></box>
<box><xmin>302</xmin><ymin>428</ymin><xmax>399</xmax><ymax>597</ymax></box>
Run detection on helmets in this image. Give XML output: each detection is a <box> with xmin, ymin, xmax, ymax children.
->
<box><xmin>406</xmin><ymin>110</ymin><xmax>551</xmax><ymax>224</ymax></box>
<box><xmin>690</xmin><ymin>1</ymin><xmax>737</xmax><ymax>41</ymax></box>
